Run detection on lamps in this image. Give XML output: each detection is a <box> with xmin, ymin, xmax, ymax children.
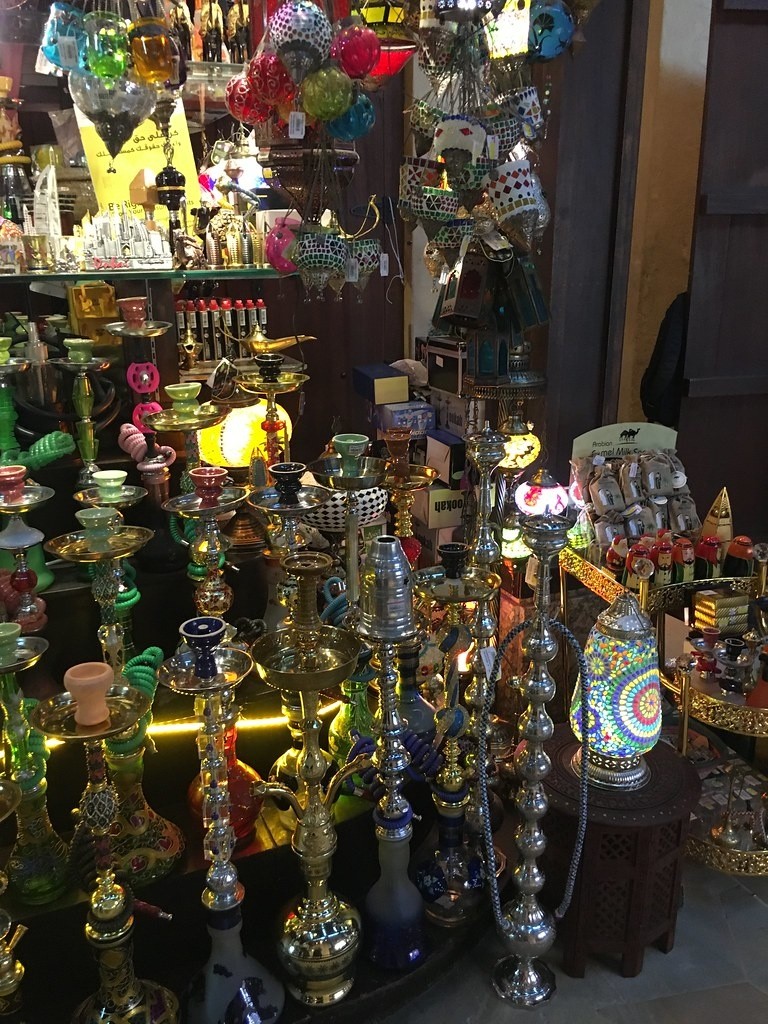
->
<box><xmin>198</xmin><ymin>371</ymin><xmax>292</xmax><ymax>547</ymax></box>
<box><xmin>569</xmin><ymin>587</ymin><xmax>664</xmax><ymax>793</ymax></box>
<box><xmin>350</xmin><ymin>0</ymin><xmax>576</xmax><ymax>438</ymax></box>
<box><xmin>491</xmin><ymin>403</ymin><xmax>541</xmax><ymax>558</ymax></box>
<box><xmin>515</xmin><ymin>469</ymin><xmax>568</xmax><ymax>591</ymax></box>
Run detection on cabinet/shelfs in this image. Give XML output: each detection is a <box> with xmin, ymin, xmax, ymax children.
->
<box><xmin>560</xmin><ymin>422</ymin><xmax>768</xmax><ymax>877</ymax></box>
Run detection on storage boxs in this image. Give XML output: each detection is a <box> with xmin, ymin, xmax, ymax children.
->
<box><xmin>353</xmin><ymin>334</ymin><xmax>487</xmax><ymax>568</ymax></box>
<box><xmin>255</xmin><ymin>209</ymin><xmax>334</xmax><ymax>263</ymax></box>
<box><xmin>66</xmin><ymin>286</ymin><xmax>122</xmax><ymax>347</ymax></box>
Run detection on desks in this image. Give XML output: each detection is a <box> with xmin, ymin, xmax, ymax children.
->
<box><xmin>533</xmin><ymin>722</ymin><xmax>702</xmax><ymax>980</ymax></box>
